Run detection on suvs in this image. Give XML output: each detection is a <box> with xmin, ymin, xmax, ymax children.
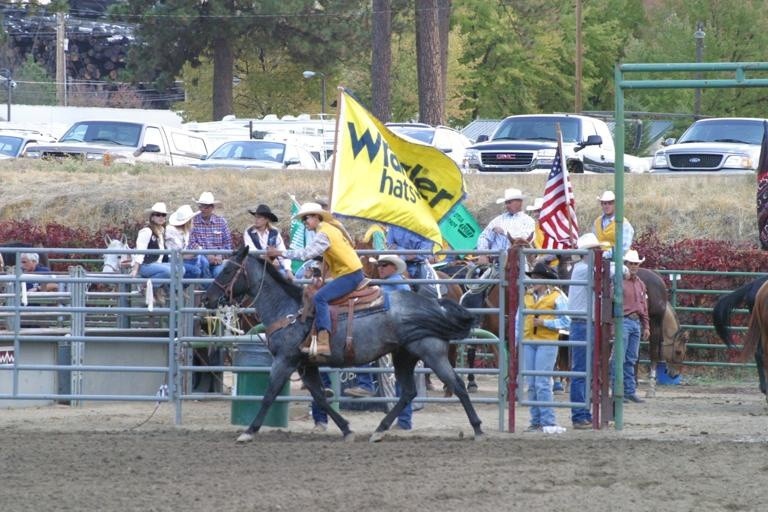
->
<box><xmin>326</xmin><ymin>123</ymin><xmax>473</xmax><ymax>173</ymax></box>
<box><xmin>0</xmin><ymin>127</ymin><xmax>56</xmax><ymax>161</ymax></box>
<box><xmin>653</xmin><ymin>118</ymin><xmax>768</xmax><ymax>172</ymax></box>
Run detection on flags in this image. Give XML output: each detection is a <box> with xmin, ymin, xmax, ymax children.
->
<box><xmin>330</xmin><ymin>88</ymin><xmax>470</xmax><ymax>257</ymax></box>
<box><xmin>536</xmin><ymin>145</ymin><xmax>579</xmax><ymax>256</ymax></box>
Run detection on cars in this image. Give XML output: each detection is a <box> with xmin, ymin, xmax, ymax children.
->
<box><xmin>186</xmin><ymin>138</ymin><xmax>322</xmax><ymax>171</ymax></box>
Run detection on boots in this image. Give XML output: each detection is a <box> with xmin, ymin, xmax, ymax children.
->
<box><xmin>301</xmin><ymin>329</ymin><xmax>331</xmax><ymax>356</ymax></box>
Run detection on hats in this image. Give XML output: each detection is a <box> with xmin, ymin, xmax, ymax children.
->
<box><xmin>368</xmin><ymin>254</ymin><xmax>407</xmax><ymax>273</ymax></box>
<box><xmin>496</xmin><ymin>188</ymin><xmax>645</xmax><ymax>279</ymax></box>
<box><xmin>152</xmin><ymin>192</ymin><xmax>332</xmax><ymax>225</ymax></box>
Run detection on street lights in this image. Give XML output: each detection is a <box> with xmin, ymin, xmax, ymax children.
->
<box><xmin>303</xmin><ymin>70</ymin><xmax>327</xmax><ymax>121</ymax></box>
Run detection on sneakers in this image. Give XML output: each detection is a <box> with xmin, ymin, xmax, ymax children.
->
<box><xmin>523</xmin><ymin>382</ymin><xmax>646</xmax><ymax>434</ymax></box>
<box><xmin>344</xmin><ymin>386</ymin><xmax>372</xmax><ymax>397</ymax></box>
<box><xmin>311</xmin><ymin>422</ymin><xmax>327</xmax><ymax>435</ymax></box>
<box><xmin>325</xmin><ymin>388</ymin><xmax>335</xmax><ymax>397</ymax></box>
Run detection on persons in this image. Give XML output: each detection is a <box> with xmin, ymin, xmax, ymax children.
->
<box><xmin>344</xmin><ymin>255</ymin><xmax>412</xmax><ymax>429</ymax></box>
<box><xmin>512</xmin><ymin>261</ymin><xmax>571</xmax><ymax>433</ymax></box>
<box><xmin>603</xmin><ymin>249</ymin><xmax>651</xmax><ymax>404</ymax></box>
<box><xmin>527</xmin><ymin>197</ymin><xmax>560</xmax><ymax>277</ymax></box>
<box><xmin>567</xmin><ymin>231</ymin><xmax>630</xmax><ymax>429</ymax></box>
<box><xmin>589</xmin><ymin>189</ymin><xmax>634</xmax><ymax>262</ymax></box>
<box><xmin>126</xmin><ymin>190</ymin><xmax>438</xmax><ymax>292</ymax></box>
<box><xmin>295</xmin><ymin>258</ymin><xmax>330</xmax><ymax>436</ymax></box>
<box><xmin>475</xmin><ymin>188</ymin><xmax>536</xmax><ymax>304</ymax></box>
<box><xmin>268</xmin><ymin>203</ymin><xmax>364</xmax><ymax>357</ymax></box>
<box><xmin>405</xmin><ymin>109</ymin><xmax>420</xmax><ymax>123</ymax></box>
<box><xmin>11</xmin><ymin>251</ymin><xmax>59</xmax><ymax>329</ymax></box>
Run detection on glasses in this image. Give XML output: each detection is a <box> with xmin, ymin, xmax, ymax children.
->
<box><xmin>376</xmin><ymin>262</ymin><xmax>392</xmax><ymax>267</ymax></box>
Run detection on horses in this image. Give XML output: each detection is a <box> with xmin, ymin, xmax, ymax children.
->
<box><xmin>200</xmin><ymin>244</ymin><xmax>489</xmax><ymax>443</ymax></box>
<box><xmin>102</xmin><ymin>233</ymin><xmax>143</xmax><ymax>289</ymax></box>
<box><xmin>240</xmin><ymin>293</ymin><xmax>262</xmax><ymax>336</ymax></box>
<box><xmin>552</xmin><ymin>252</ymin><xmax>666</xmax><ymax>398</ymax></box>
<box><xmin>737</xmin><ymin>280</ymin><xmax>768</xmax><ymax>403</ymax></box>
<box><xmin>712</xmin><ymin>276</ymin><xmax>768</xmax><ymax>395</ymax></box>
<box><xmin>483</xmin><ymin>231</ymin><xmax>538</xmax><ymax>341</ymax></box>
<box><xmin>424</xmin><ymin>263</ymin><xmax>501</xmax><ymax>393</ymax></box>
<box><xmin>661</xmin><ymin>299</ymin><xmax>690</xmax><ymax>379</ymax></box>
<box><xmin>354</xmin><ymin>237</ymin><xmax>464</xmax><ymax>398</ymax></box>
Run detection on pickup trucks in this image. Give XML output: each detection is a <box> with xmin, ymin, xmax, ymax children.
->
<box><xmin>22</xmin><ymin>120</ymin><xmax>209</xmax><ymax>165</ymax></box>
<box><xmin>467</xmin><ymin>112</ymin><xmax>643</xmax><ymax>174</ymax></box>
